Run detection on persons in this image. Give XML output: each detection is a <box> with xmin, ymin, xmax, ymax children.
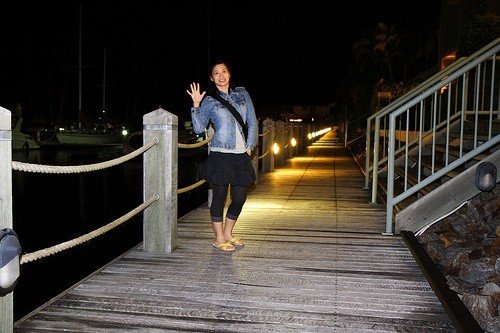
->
<box><xmin>186</xmin><ymin>60</ymin><xmax>258</xmax><ymax>251</ymax></box>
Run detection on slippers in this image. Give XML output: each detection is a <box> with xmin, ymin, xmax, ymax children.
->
<box><xmin>213</xmin><ymin>240</ymin><xmax>236</xmax><ymax>252</ymax></box>
<box><xmin>221</xmin><ymin>234</ymin><xmax>246</xmax><ymax>246</ymax></box>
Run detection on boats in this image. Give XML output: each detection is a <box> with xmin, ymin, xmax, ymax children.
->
<box><xmin>56</xmin><ymin>123</ymin><xmax>126</xmax><ymax>145</ymax></box>
<box><xmin>12</xmin><ymin>117</ymin><xmax>41</xmax><ymax>150</ymax></box>
